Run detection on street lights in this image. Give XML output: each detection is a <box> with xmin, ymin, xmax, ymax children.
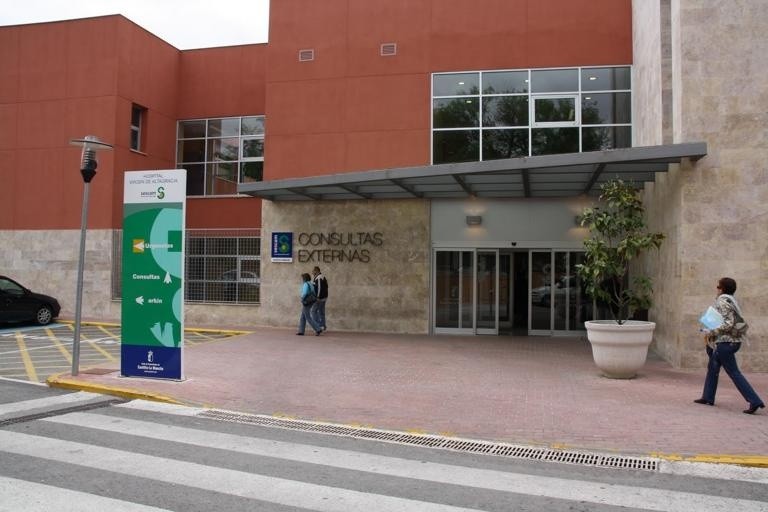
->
<box><xmin>69</xmin><ymin>134</ymin><xmax>114</xmax><ymax>376</ymax></box>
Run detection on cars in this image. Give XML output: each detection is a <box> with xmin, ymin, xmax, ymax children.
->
<box><xmin>218</xmin><ymin>268</ymin><xmax>260</xmax><ymax>291</ymax></box>
<box><xmin>0</xmin><ymin>275</ymin><xmax>61</xmax><ymax>328</ymax></box>
<box><xmin>532</xmin><ymin>274</ymin><xmax>590</xmax><ymax>308</ymax></box>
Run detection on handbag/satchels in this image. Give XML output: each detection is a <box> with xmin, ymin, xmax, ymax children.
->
<box><xmin>730</xmin><ymin>313</ymin><xmax>748</xmax><ymax>338</ymax></box>
<box><xmin>303</xmin><ymin>290</ymin><xmax>317</xmax><ymax>307</ymax></box>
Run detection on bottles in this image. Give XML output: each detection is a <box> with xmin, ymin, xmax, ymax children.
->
<box><xmin>699</xmin><ymin>328</ymin><xmax>710</xmax><ymax>333</ymax></box>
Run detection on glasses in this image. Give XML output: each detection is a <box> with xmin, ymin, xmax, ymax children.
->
<box><xmin>717</xmin><ymin>286</ymin><xmax>722</xmax><ymax>289</ymax></box>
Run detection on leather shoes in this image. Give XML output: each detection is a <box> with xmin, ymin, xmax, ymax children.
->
<box><xmin>296</xmin><ymin>332</ymin><xmax>304</xmax><ymax>335</ymax></box>
<box><xmin>315</xmin><ymin>326</ymin><xmax>327</xmax><ymax>336</ymax></box>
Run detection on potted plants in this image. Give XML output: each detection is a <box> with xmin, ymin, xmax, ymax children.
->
<box><xmin>570</xmin><ymin>179</ymin><xmax>668</xmax><ymax>381</ymax></box>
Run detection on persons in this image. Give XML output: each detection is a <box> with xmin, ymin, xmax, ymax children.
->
<box><xmin>295</xmin><ymin>266</ymin><xmax>328</xmax><ymax>336</ymax></box>
<box><xmin>694</xmin><ymin>277</ymin><xmax>765</xmax><ymax>414</ymax></box>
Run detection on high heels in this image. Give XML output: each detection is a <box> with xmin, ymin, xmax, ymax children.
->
<box><xmin>694</xmin><ymin>397</ymin><xmax>714</xmax><ymax>405</ymax></box>
<box><xmin>744</xmin><ymin>402</ymin><xmax>765</xmax><ymax>414</ymax></box>
